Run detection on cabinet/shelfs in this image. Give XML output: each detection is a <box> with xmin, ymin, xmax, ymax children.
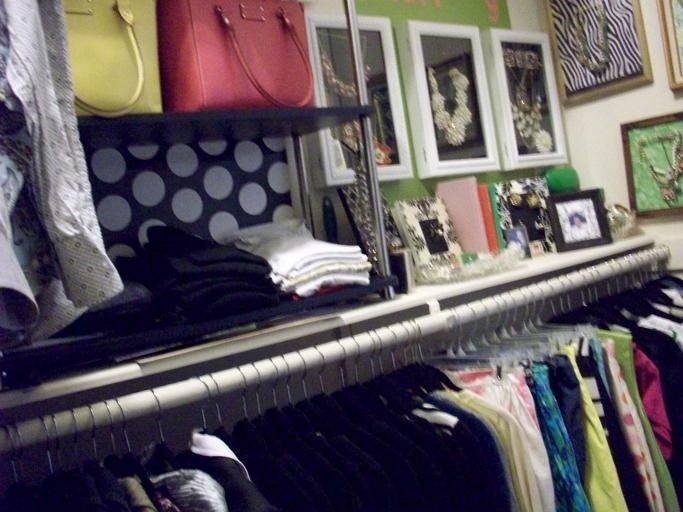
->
<box><xmin>1</xmin><ymin>0</ymin><xmax>398</xmax><ymax>388</ymax></box>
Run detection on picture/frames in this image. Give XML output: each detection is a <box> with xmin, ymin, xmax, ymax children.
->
<box><xmin>544</xmin><ymin>0</ymin><xmax>683</xmax><ymax>105</ymax></box>
<box><xmin>547</xmin><ymin>189</ymin><xmax>613</xmax><ymax>254</ymax></box>
<box><xmin>620</xmin><ymin>111</ymin><xmax>683</xmax><ymax>219</ymax></box>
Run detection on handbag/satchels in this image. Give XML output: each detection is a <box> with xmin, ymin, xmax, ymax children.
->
<box><xmin>157</xmin><ymin>0</ymin><xmax>315</xmax><ymax>113</ymax></box>
<box><xmin>61</xmin><ymin>0</ymin><xmax>165</xmax><ymax>119</ymax></box>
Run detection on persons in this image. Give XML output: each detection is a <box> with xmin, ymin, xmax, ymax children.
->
<box><xmin>570</xmin><ymin>213</ymin><xmax>588</xmax><ymax>234</ymax></box>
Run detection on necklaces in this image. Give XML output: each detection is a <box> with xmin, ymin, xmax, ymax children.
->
<box><xmin>429</xmin><ymin>65</ymin><xmax>474</xmax><ymax>146</ymax></box>
<box><xmin>575</xmin><ymin>0</ymin><xmax>609</xmax><ymax>73</ymax></box>
<box><xmin>337</xmin><ymin>98</ymin><xmax>394</xmax><ymax>167</ymax></box>
<box><xmin>505</xmin><ymin>47</ymin><xmax>553</xmax><ymax>154</ymax></box>
<box><xmin>637</xmin><ymin>129</ymin><xmax>683</xmax><ymax>201</ymax></box>
<box><xmin>319</xmin><ymin>29</ymin><xmax>371</xmax><ymax>97</ymax></box>
